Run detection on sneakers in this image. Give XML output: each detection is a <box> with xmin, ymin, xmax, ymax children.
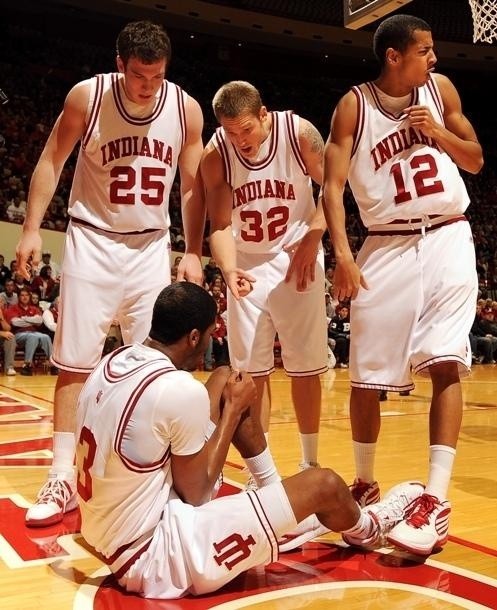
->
<box><xmin>24</xmin><ymin>469</ymin><xmax>78</xmax><ymax>526</ymax></box>
<box><xmin>278</xmin><ymin>479</ymin><xmax>452</xmax><ymax>556</ymax></box>
<box><xmin>4</xmin><ymin>358</ymin><xmax>59</xmax><ymax>376</ymax></box>
<box><xmin>472</xmin><ymin>353</ymin><xmax>496</xmax><ymax>364</ymax></box>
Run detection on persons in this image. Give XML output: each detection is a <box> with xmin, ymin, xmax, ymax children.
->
<box><xmin>169</xmin><ymin>182</ymin><xmax>184</xmax><ymax>252</ymax></box>
<box><xmin>321</xmin><ymin>14</ymin><xmax>485</xmax><ymax>553</ymax></box>
<box><xmin>1</xmin><ymin>104</ymin><xmax>78</xmax><ymax>232</ymax></box>
<box><xmin>1</xmin><ymin>249</ymin><xmax>60</xmax><ymax>376</ymax></box>
<box><xmin>198</xmin><ymin>78</ymin><xmax>326</xmax><ymax>488</ymax></box>
<box><xmin>323</xmin><ymin>184</ymin><xmax>367</xmax><ymax>368</ymax></box>
<box><xmin>68</xmin><ymin>280</ymin><xmax>427</xmax><ymax>599</ymax></box>
<box><xmin>12</xmin><ymin>18</ymin><xmax>204</xmax><ymax>528</ymax></box>
<box><xmin>171</xmin><ymin>256</ymin><xmax>229</xmax><ymax>371</ymax></box>
<box><xmin>463</xmin><ymin>173</ymin><xmax>497</xmax><ymax>365</ymax></box>
